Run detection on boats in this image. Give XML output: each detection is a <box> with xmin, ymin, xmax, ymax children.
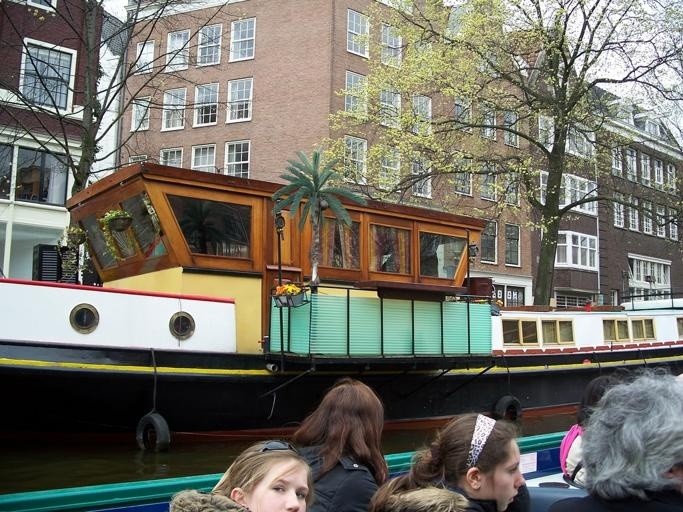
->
<box><xmin>0</xmin><ymin>161</ymin><xmax>683</xmax><ymax>446</ymax></box>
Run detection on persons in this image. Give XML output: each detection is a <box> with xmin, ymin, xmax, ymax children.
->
<box><xmin>548</xmin><ymin>363</ymin><xmax>682</xmax><ymax>512</ymax></box>
<box><xmin>367</xmin><ymin>411</ymin><xmax>525</xmax><ymax>512</ymax></box>
<box><xmin>558</xmin><ymin>375</ymin><xmax>624</xmax><ymax>491</ymax></box>
<box><xmin>286</xmin><ymin>377</ymin><xmax>387</xmax><ymax>512</ymax></box>
<box><xmin>481</xmin><ymin>411</ymin><xmax>530</xmax><ymax>512</ymax></box>
<box><xmin>168</xmin><ymin>437</ymin><xmax>315</xmax><ymax>512</ymax></box>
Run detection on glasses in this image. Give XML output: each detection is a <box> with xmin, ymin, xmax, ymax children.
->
<box><xmin>255</xmin><ymin>440</ymin><xmax>300</xmax><ymax>456</ymax></box>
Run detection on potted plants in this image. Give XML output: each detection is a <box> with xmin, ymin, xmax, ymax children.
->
<box><xmin>270</xmin><ymin>284</ymin><xmax>303</xmax><ymax>308</ymax></box>
<box><xmin>94</xmin><ymin>209</ymin><xmax>132</xmax><ymax>261</ymax></box>
<box><xmin>54</xmin><ymin>227</ymin><xmax>90</xmax><ymax>272</ymax></box>
